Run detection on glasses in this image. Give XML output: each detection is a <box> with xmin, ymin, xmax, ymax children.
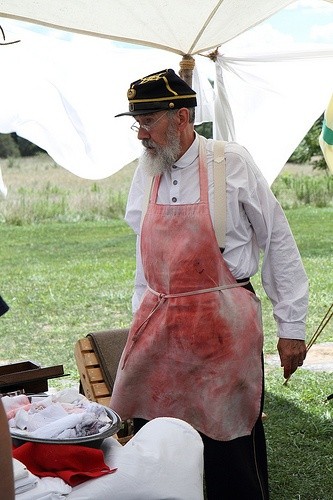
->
<box><xmin>131</xmin><ymin>111</ymin><xmax>168</xmax><ymax>133</ymax></box>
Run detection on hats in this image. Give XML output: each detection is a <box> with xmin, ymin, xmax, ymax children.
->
<box><xmin>115</xmin><ymin>69</ymin><xmax>197</xmax><ymax>117</ymax></box>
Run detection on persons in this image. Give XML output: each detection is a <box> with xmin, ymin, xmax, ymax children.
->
<box><xmin>107</xmin><ymin>68</ymin><xmax>310</xmax><ymax>500</ymax></box>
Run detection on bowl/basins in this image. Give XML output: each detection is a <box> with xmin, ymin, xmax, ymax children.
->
<box><xmin>10</xmin><ymin>395</ymin><xmax>122</xmax><ymax>450</ymax></box>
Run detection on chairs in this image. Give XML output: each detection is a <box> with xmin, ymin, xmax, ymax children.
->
<box><xmin>74</xmin><ymin>328</ymin><xmax>134</xmax><ymax>445</ymax></box>
